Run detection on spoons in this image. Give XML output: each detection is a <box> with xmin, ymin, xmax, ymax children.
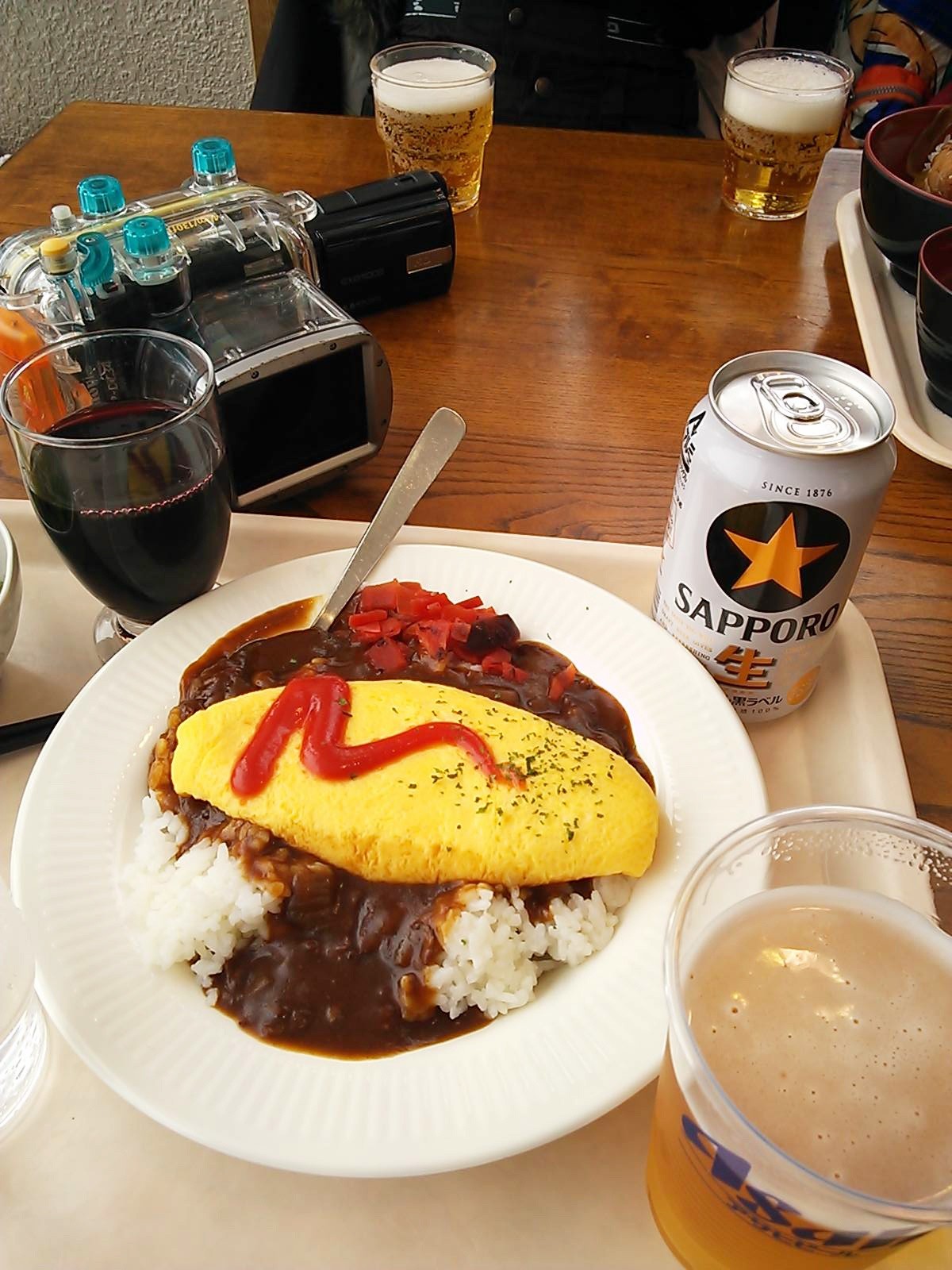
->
<box><xmin>224</xmin><ymin>406</ymin><xmax>470</xmax><ymax>679</ymax></box>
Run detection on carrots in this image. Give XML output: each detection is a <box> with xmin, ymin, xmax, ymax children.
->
<box><xmin>344</xmin><ymin>581</ymin><xmax>579</xmax><ymax>701</ymax></box>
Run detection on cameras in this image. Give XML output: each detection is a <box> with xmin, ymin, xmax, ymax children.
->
<box><xmin>0</xmin><ymin>141</ymin><xmax>461</xmax><ymax>511</ymax></box>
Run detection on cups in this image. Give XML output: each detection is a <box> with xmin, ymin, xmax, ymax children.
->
<box><xmin>720</xmin><ymin>47</ymin><xmax>853</xmax><ymax>222</ymax></box>
<box><xmin>370</xmin><ymin>41</ymin><xmax>497</xmax><ymax>216</ymax></box>
<box><xmin>642</xmin><ymin>804</ymin><xmax>952</xmax><ymax>1270</ymax></box>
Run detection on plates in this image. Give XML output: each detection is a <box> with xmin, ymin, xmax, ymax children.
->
<box><xmin>10</xmin><ymin>544</ymin><xmax>769</xmax><ymax>1182</ymax></box>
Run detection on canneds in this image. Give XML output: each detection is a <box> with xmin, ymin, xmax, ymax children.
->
<box><xmin>649</xmin><ymin>348</ymin><xmax>896</xmax><ymax>728</ymax></box>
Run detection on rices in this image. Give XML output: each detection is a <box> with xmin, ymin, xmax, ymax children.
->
<box><xmin>126</xmin><ymin>796</ymin><xmax>623</xmax><ymax>1023</ymax></box>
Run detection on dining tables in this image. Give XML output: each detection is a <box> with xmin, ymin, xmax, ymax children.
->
<box><xmin>0</xmin><ymin>100</ymin><xmax>952</xmax><ymax>932</ymax></box>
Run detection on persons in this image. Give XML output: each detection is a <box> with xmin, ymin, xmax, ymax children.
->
<box><xmin>250</xmin><ymin>0</ymin><xmax>711</xmax><ymax>138</ymax></box>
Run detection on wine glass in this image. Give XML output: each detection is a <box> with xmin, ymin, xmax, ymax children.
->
<box><xmin>0</xmin><ymin>331</ymin><xmax>232</xmax><ymax>669</ymax></box>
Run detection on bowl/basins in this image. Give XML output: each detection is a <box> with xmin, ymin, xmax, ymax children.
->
<box><xmin>859</xmin><ymin>105</ymin><xmax>952</xmax><ymax>295</ymax></box>
<box><xmin>914</xmin><ymin>226</ymin><xmax>952</xmax><ymax>419</ymax></box>
